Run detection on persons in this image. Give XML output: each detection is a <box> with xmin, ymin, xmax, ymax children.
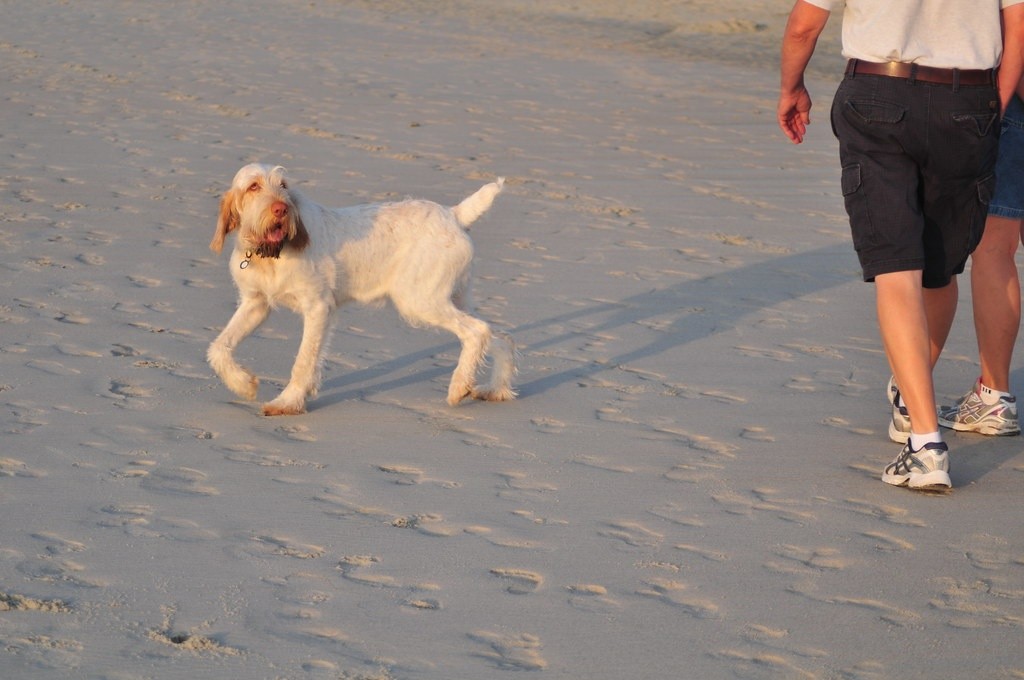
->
<box><xmin>778</xmin><ymin>0</ymin><xmax>1024</xmax><ymax>489</ymax></box>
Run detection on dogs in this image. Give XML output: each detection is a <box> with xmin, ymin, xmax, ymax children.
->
<box><xmin>205</xmin><ymin>163</ymin><xmax>519</xmax><ymax>418</ymax></box>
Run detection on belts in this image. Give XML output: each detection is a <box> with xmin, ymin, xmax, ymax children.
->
<box><xmin>844</xmin><ymin>58</ymin><xmax>997</xmax><ymax>85</ymax></box>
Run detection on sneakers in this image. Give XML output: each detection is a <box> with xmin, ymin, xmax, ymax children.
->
<box><xmin>887</xmin><ymin>375</ymin><xmax>912</xmax><ymax>443</ymax></box>
<box><xmin>881</xmin><ymin>437</ymin><xmax>952</xmax><ymax>492</ymax></box>
<box><xmin>936</xmin><ymin>376</ymin><xmax>1021</xmax><ymax>435</ymax></box>
